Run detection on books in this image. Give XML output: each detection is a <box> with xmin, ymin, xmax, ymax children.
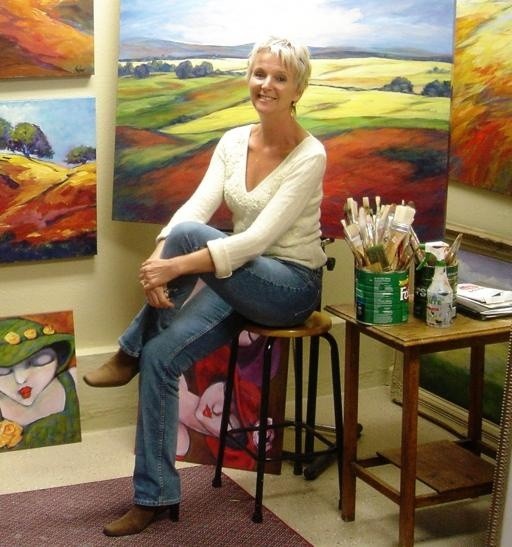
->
<box><xmin>457</xmin><ymin>283</ymin><xmax>512</xmax><ymax>321</ymax></box>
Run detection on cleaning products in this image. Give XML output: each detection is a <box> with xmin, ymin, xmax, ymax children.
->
<box><xmin>422</xmin><ymin>241</ymin><xmax>454</xmax><ymax>328</ymax></box>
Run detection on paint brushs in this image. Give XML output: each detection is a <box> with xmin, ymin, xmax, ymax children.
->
<box><xmin>410</xmin><ymin>226</ymin><xmax>462</xmax><ymax>265</ymax></box>
<box><xmin>341</xmin><ymin>194</ymin><xmax>416</xmax><ymax>273</ymax></box>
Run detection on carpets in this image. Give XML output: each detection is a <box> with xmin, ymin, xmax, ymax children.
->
<box><xmin>3</xmin><ymin>463</ymin><xmax>313</xmax><ymax>545</ymax></box>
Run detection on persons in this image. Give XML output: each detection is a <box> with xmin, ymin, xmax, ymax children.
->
<box><xmin>175</xmin><ymin>344</ymin><xmax>262</xmax><ymax>469</ymax></box>
<box><xmin>1</xmin><ymin>316</ymin><xmax>82</xmax><ymax>449</ymax></box>
<box><xmin>83</xmin><ymin>35</ymin><xmax>327</xmax><ymax>536</ymax></box>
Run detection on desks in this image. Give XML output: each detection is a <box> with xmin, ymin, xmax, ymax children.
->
<box><xmin>326</xmin><ymin>301</ymin><xmax>511</xmax><ymax>547</ymax></box>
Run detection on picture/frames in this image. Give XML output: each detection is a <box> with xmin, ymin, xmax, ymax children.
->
<box><xmin>388</xmin><ymin>231</ymin><xmax>512</xmax><ymax>452</ymax></box>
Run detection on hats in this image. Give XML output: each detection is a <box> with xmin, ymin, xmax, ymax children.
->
<box><xmin>0</xmin><ymin>318</ymin><xmax>75</xmax><ymax>375</ymax></box>
<box><xmin>193</xmin><ymin>354</ymin><xmax>266</xmax><ymax>470</ymax></box>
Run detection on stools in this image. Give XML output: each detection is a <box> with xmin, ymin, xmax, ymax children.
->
<box><xmin>202</xmin><ymin>311</ymin><xmax>343</xmax><ymax>522</ymax></box>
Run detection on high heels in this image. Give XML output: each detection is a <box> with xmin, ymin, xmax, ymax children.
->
<box><xmin>83</xmin><ymin>349</ymin><xmax>140</xmax><ymax>387</ymax></box>
<box><xmin>103</xmin><ymin>504</ymin><xmax>180</xmax><ymax>536</ymax></box>
